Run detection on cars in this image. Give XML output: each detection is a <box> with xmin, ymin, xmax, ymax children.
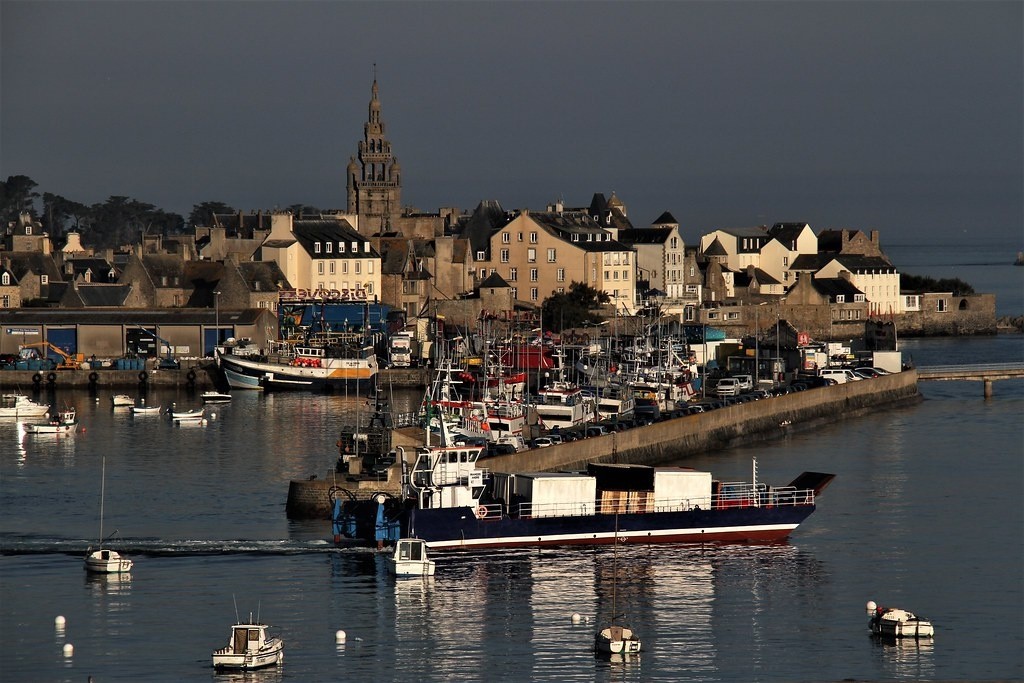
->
<box><xmin>855</xmin><ymin>366</ymin><xmax>892</xmax><ymax>378</ymax></box>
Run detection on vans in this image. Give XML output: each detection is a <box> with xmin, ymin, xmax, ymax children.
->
<box><xmin>819</xmin><ymin>368</ymin><xmax>863</xmax><ymax>383</ymax></box>
<box><xmin>732</xmin><ymin>374</ymin><xmax>753</xmax><ymax>394</ymax></box>
<box><xmin>715</xmin><ymin>377</ymin><xmax>742</xmax><ymax>398</ymax></box>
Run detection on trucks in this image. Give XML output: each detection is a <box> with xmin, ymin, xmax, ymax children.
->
<box><xmin>389</xmin><ymin>335</ymin><xmax>413</xmax><ymax>368</ymax></box>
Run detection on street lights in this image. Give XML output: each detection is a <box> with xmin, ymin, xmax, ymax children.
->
<box><xmin>754</xmin><ymin>301</ymin><xmax>768</xmax><ymax>383</ymax></box>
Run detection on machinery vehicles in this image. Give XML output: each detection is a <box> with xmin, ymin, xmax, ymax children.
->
<box><xmin>137</xmin><ymin>324</ymin><xmax>180</xmax><ymax>370</ymax></box>
<box><xmin>18</xmin><ymin>341</ymin><xmax>82</xmax><ymax>370</ymax></box>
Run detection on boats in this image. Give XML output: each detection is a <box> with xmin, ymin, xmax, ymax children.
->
<box><xmin>383</xmin><ymin>537</ymin><xmax>435</xmax><ymax>577</ymax></box>
<box><xmin>166</xmin><ymin>407</ymin><xmax>205</xmax><ymax>417</ymax></box>
<box><xmin>199</xmin><ymin>391</ymin><xmax>232</xmax><ymax>404</ymax></box>
<box><xmin>83</xmin><ymin>454</ymin><xmax>133</xmax><ymax>572</ymax></box>
<box><xmin>22</xmin><ymin>400</ymin><xmax>79</xmax><ymax>434</ymax></box>
<box><xmin>0</xmin><ymin>382</ymin><xmax>52</xmax><ymax>417</ymax></box>
<box><xmin>595</xmin><ymin>509</ymin><xmax>642</xmax><ymax>655</ymax></box>
<box><xmin>212</xmin><ymin>593</ymin><xmax>285</xmax><ymax>669</ymax></box>
<box><xmin>129</xmin><ymin>405</ymin><xmax>161</xmax><ymax>413</ymax></box>
<box><xmin>412</xmin><ymin>295</ymin><xmax>704</xmax><ymax>454</ymax></box>
<box><xmin>110</xmin><ymin>392</ymin><xmax>134</xmax><ymax>405</ymax></box>
<box><xmin>865</xmin><ymin>601</ymin><xmax>934</xmax><ymax>638</ymax></box>
<box><xmin>327</xmin><ymin>412</ymin><xmax>836</xmax><ymax>555</ymax></box>
<box><xmin>217</xmin><ymin>330</ymin><xmax>379</xmax><ymax>391</ymax></box>
